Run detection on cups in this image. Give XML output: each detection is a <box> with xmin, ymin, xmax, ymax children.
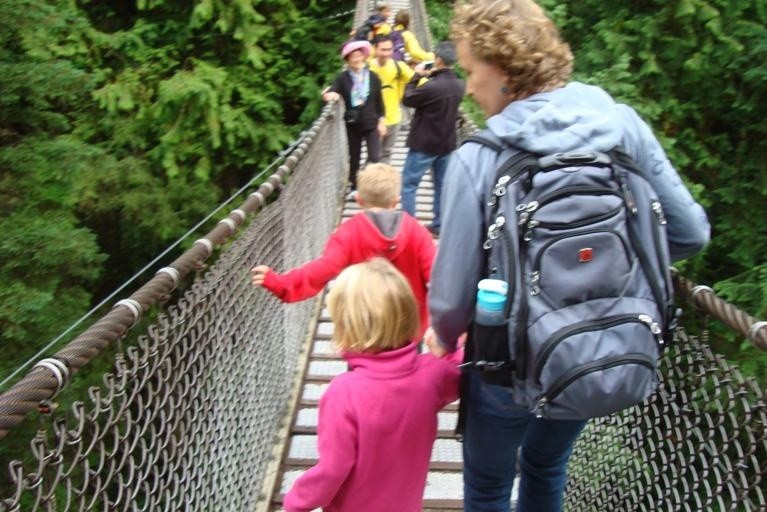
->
<box><xmin>470</xmin><ymin>279</ymin><xmax>511</xmax><ymax>325</ymax></box>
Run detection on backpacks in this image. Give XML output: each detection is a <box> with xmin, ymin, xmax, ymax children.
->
<box><xmin>455</xmin><ymin>127</ymin><xmax>677</xmax><ymax>420</ymax></box>
<box><xmin>356</xmin><ymin>20</ymin><xmax>407</xmax><ymax>62</ymax></box>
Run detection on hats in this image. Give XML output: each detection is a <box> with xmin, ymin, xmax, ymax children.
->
<box><xmin>340</xmin><ymin>37</ymin><xmax>372</xmax><ymax>59</ymax></box>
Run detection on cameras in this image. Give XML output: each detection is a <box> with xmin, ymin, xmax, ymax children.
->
<box><xmin>424</xmin><ymin>61</ymin><xmax>434</xmax><ymax>70</ymax></box>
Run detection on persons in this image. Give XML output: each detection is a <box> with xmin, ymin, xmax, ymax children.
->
<box><xmin>279</xmin><ymin>258</ymin><xmax>467</xmax><ymax>511</ymax></box>
<box><xmin>321</xmin><ymin>2</ymin><xmax>466</xmax><ymax>238</ymax></box>
<box><xmin>252</xmin><ymin>161</ymin><xmax>438</xmax><ymax>354</ymax></box>
<box><xmin>423</xmin><ymin>0</ymin><xmax>710</xmax><ymax>512</ymax></box>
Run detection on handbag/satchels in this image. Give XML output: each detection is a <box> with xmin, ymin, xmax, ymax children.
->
<box><xmin>345</xmin><ymin>109</ymin><xmax>362</xmax><ymax>135</ymax></box>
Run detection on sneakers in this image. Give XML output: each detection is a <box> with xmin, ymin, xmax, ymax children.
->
<box><xmin>345</xmin><ymin>190</ymin><xmax>356</xmax><ymax>201</ymax></box>
<box><xmin>424</xmin><ymin>223</ymin><xmax>440</xmax><ymax>239</ymax></box>
<box><xmin>400</xmin><ymin>123</ymin><xmax>412</xmax><ymax>131</ymax></box>
